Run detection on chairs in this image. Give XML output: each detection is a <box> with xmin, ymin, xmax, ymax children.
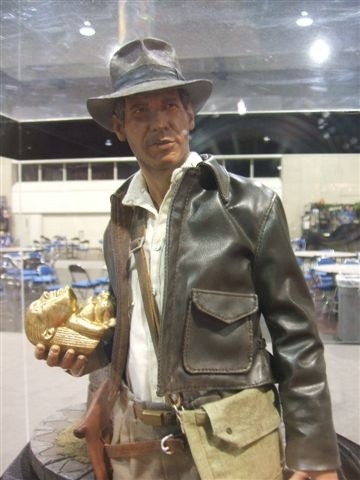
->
<box><xmin>0</xmin><ymin>234</ymin><xmax>359</xmax><ymax>320</ymax></box>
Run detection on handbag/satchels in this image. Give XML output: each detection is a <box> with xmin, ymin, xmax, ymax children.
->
<box><xmin>169</xmin><ymin>386</ymin><xmax>287</xmax><ymax>480</ymax></box>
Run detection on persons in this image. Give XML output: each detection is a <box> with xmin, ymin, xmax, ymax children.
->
<box><xmin>35</xmin><ymin>37</ymin><xmax>342</xmax><ymax>480</ymax></box>
<box><xmin>26</xmin><ymin>285</ymin><xmax>118</xmax><ymax>355</ymax></box>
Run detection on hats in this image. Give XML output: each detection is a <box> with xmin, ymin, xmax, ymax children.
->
<box><xmin>87</xmin><ymin>37</ymin><xmax>213</xmax><ymax>133</ymax></box>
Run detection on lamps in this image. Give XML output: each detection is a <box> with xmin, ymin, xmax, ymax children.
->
<box><xmin>105</xmin><ymin>139</ymin><xmax>112</xmax><ymax>146</ymax></box>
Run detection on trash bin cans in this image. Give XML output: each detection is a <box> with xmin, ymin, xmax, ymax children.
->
<box><xmin>334</xmin><ymin>273</ymin><xmax>360</xmax><ymax>344</ymax></box>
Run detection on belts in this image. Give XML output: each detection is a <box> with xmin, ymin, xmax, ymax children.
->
<box><xmin>110</xmin><ymin>389</ymin><xmax>180</xmax><ymax>427</ymax></box>
